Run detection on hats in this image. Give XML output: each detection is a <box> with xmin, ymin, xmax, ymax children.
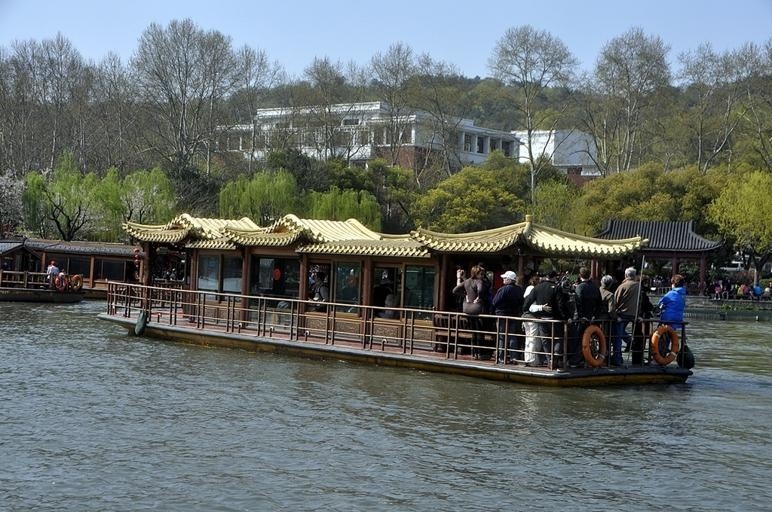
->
<box><xmin>546</xmin><ymin>271</ymin><xmax>562</xmax><ymax>279</ymax></box>
<box><xmin>500</xmin><ymin>270</ymin><xmax>516</xmax><ymax>281</ymax></box>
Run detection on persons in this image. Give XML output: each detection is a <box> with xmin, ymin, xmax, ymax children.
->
<box><xmin>273</xmin><ymin>264</ymin><xmax>432</xmax><ymax>319</ymax></box>
<box><xmin>164</xmin><ymin>269</ymin><xmax>171</xmax><ymax>278</ymax></box>
<box><xmin>170</xmin><ymin>267</ymin><xmax>178</xmax><ymax>290</ymax></box>
<box><xmin>652</xmin><ymin>273</ymin><xmax>771</xmax><ymax>300</ymax></box>
<box><xmin>47</xmin><ymin>259</ymin><xmax>60</xmax><ymax>289</ymax></box>
<box><xmin>452</xmin><ymin>261</ymin><xmax>685</xmax><ymax>369</ymax></box>
<box><xmin>59</xmin><ymin>268</ymin><xmax>66</xmax><ymax>286</ymax></box>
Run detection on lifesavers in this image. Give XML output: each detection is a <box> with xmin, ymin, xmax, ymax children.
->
<box><xmin>583</xmin><ymin>325</ymin><xmax>606</xmax><ymax>366</ymax></box>
<box><xmin>71</xmin><ymin>274</ymin><xmax>83</xmax><ymax>290</ymax></box>
<box><xmin>55</xmin><ymin>275</ymin><xmax>68</xmax><ymax>291</ymax></box>
<box><xmin>650</xmin><ymin>325</ymin><xmax>679</xmax><ymax>365</ymax></box>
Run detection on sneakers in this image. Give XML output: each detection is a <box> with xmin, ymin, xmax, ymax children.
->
<box><xmin>498</xmin><ymin>358</ymin><xmax>580</xmax><ymax>372</ymax></box>
<box><xmin>626</xmin><ymin>341</ymin><xmax>636</xmax><ymax>351</ymax></box>
